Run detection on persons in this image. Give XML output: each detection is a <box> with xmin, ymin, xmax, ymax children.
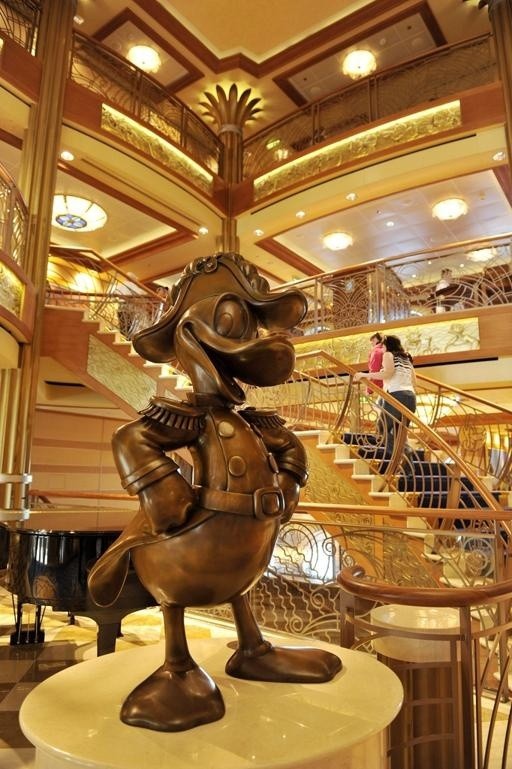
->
<box><xmin>351</xmin><ymin>334</ymin><xmax>418</xmax><ymax>474</ymax></box>
<box><xmin>435</xmin><ymin>267</ymin><xmax>463</xmax><ymax>313</ymax></box>
<box><xmin>365</xmin><ymin>332</ymin><xmax>385</xmax><ymax>407</ymax></box>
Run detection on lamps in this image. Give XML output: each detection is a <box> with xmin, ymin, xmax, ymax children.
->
<box><xmin>51</xmin><ymin>193</ymin><xmax>108</xmax><ymax>232</ymax></box>
<box><xmin>322</xmin><ymin>230</ymin><xmax>353</xmax><ymax>251</ymax></box>
<box><xmin>433</xmin><ymin>198</ymin><xmax>468</xmax><ymax>221</ymax></box>
<box><xmin>343</xmin><ymin>50</ymin><xmax>376</xmax><ymax>81</ymax></box>
<box><xmin>126</xmin><ymin>45</ymin><xmax>161</xmax><ymax>73</ymax></box>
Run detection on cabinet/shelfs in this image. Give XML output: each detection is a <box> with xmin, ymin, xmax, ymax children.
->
<box><xmin>18</xmin><ymin>636</ymin><xmax>406</xmax><ymax>769</ymax></box>
